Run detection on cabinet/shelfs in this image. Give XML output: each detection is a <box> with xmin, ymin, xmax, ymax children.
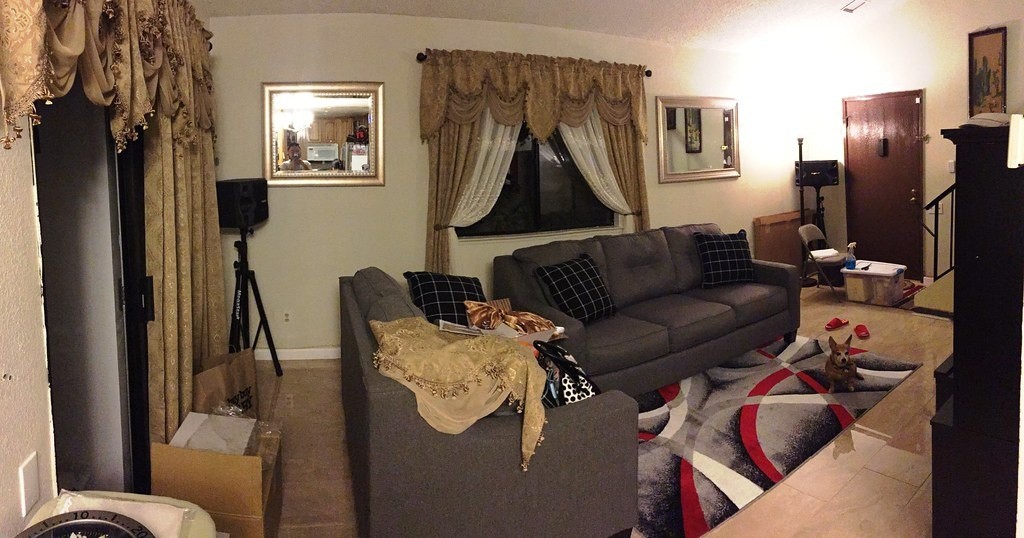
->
<box><xmin>929</xmin><ymin>126</ymin><xmax>1024</xmax><ymax>538</ymax></box>
<box><xmin>302</xmin><ymin>115</ymin><xmax>355</xmax><ymax>144</ymax></box>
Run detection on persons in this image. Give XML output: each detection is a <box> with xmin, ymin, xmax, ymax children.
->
<box><xmin>277</xmin><ymin>142</ymin><xmax>312</xmax><ymax>170</ymax></box>
<box><xmin>331</xmin><ymin>159</ymin><xmax>343</xmax><ymax>171</ymax></box>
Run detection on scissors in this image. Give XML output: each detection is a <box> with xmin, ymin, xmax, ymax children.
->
<box><xmin>861</xmin><ymin>263</ymin><xmax>872</xmax><ymax>270</ymax></box>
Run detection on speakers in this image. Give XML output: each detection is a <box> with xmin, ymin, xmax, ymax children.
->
<box><xmin>794</xmin><ymin>160</ymin><xmax>839</xmax><ymax>185</ymax></box>
<box><xmin>216</xmin><ymin>178</ymin><xmax>269</xmax><ymax>230</ymax></box>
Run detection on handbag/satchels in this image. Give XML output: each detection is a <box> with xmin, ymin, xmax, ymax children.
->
<box><xmin>194</xmin><ymin>347</ymin><xmax>260</xmax><ymax>420</ymax></box>
<box><xmin>533</xmin><ymin>340</ymin><xmax>601</xmax><ymax>409</ymax></box>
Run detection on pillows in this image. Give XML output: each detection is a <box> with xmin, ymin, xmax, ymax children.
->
<box><xmin>536</xmin><ymin>254</ymin><xmax>617</xmax><ymax>326</ymax></box>
<box><xmin>403</xmin><ymin>272</ymin><xmax>486</xmax><ymax>326</ymax></box>
<box><xmin>693</xmin><ymin>229</ymin><xmax>757</xmax><ymax>289</ymax></box>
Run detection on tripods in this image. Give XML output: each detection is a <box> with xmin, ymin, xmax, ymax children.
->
<box><xmin>808</xmin><ymin>186</ymin><xmax>827</xmax><ymax>250</ymax></box>
<box><xmin>227</xmin><ymin>228</ymin><xmax>284</xmax><ymax>376</ymax></box>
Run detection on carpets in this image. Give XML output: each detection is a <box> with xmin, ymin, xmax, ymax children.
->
<box><xmin>897</xmin><ymin>282</ymin><xmax>925</xmax><ymax>305</ymax></box>
<box><xmin>634</xmin><ymin>334</ymin><xmax>924</xmax><ymax>538</ymax></box>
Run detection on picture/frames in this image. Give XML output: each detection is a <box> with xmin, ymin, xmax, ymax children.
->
<box><xmin>968</xmin><ymin>27</ymin><xmax>1006</xmax><ymax>118</ymax></box>
<box><xmin>684</xmin><ymin>107</ymin><xmax>702</xmax><ymax>155</ymax></box>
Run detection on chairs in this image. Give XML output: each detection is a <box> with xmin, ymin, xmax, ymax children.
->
<box><xmin>798</xmin><ymin>224</ymin><xmax>849</xmax><ymax>304</ymax></box>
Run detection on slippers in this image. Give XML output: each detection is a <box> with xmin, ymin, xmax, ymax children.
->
<box><xmin>854</xmin><ymin>324</ymin><xmax>870</xmax><ymax>338</ymax></box>
<box><xmin>825</xmin><ymin>317</ymin><xmax>849</xmax><ymax>330</ymax></box>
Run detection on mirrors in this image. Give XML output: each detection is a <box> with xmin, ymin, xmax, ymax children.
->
<box><xmin>654</xmin><ymin>96</ymin><xmax>741</xmax><ymax>184</ymax></box>
<box><xmin>261</xmin><ymin>81</ymin><xmax>384</xmax><ymax>188</ymax></box>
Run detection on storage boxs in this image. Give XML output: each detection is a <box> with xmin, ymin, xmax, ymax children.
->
<box><xmin>840</xmin><ymin>260</ymin><xmax>907</xmax><ymax>307</ymax></box>
<box><xmin>150</xmin><ymin>411</ymin><xmax>283</xmax><ymax>538</ymax></box>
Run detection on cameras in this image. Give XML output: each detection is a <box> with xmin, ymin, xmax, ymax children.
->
<box><xmin>294</xmin><ymin>154</ymin><xmax>298</xmax><ymax>158</ymax></box>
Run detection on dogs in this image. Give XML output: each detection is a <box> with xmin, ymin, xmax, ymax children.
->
<box><xmin>825</xmin><ymin>334</ymin><xmax>865</xmax><ymax>394</ymax></box>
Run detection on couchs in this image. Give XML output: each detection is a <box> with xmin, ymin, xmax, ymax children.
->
<box><xmin>493</xmin><ymin>222</ymin><xmax>801</xmax><ymax>396</ymax></box>
<box><xmin>338</xmin><ymin>266</ymin><xmax>639</xmax><ymax>538</ymax></box>
